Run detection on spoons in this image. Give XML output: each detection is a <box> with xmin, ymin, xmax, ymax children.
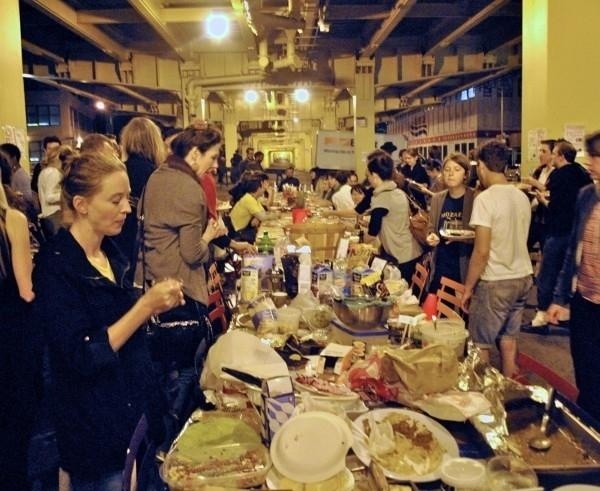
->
<box><xmin>264</xmin><ymin>398</ymin><xmax>293</xmax><ymax>434</ymax></box>
<box><xmin>527</xmin><ymin>388</ymin><xmax>557</xmax><ymax>450</ymax></box>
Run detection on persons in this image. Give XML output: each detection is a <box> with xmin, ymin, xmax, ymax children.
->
<box><xmin>0</xmin><ymin>118</ymin><xmax>300</xmax><ymax>491</ymax></box>
<box><xmin>311</xmin><ymin>119</ymin><xmax>600</xmax><ymax>423</ymax></box>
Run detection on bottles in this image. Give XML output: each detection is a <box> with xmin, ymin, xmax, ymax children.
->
<box><xmin>304</xmin><ymin>193</ymin><xmax>311</xmax><ymax>214</ymax></box>
<box><xmin>278</xmin><ymin>228</ymin><xmax>298</xmax><ymax>261</ymax></box>
<box><xmin>423</xmin><ymin>294</ymin><xmax>438</xmax><ymax>322</ymax></box>
<box><xmin>390</xmin><ymin>300</ymin><xmax>401</xmax><ymax>330</ymax></box>
<box><xmin>258</xmin><ymin>230</ymin><xmax>275</xmax><ymax>256</ymax></box>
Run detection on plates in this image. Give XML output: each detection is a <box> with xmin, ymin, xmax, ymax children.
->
<box><xmin>439</xmin><ymin>229</ymin><xmax>474</xmax><ymax>240</ymax></box>
<box><xmin>270</xmin><ymin>412</ymin><xmax>353</xmax><ymax>486</ymax></box>
<box><xmin>529</xmin><ymin>192</ymin><xmax>551</xmax><ymax>198</ymax></box>
<box><xmin>267</xmin><ymin>465</ymin><xmax>356</xmax><ymax>491</ymax></box>
<box><xmin>292</xmin><ymin>381</ymin><xmax>359</xmax><ymax>410</ymax></box>
<box><xmin>352</xmin><ymin>409</ymin><xmax>462</xmax><ymax>484</ymax></box>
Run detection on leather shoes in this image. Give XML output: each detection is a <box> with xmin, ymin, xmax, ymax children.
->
<box><xmin>520</xmin><ymin>321</ymin><xmax>549</xmax><ymax>335</ymax></box>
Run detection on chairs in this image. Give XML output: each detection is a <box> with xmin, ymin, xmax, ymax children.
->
<box><xmin>512</xmin><ymin>351</ymin><xmax>579</xmax><ymax>405</ymax></box>
<box><xmin>207</xmin><ymin>290</ymin><xmax>228</xmax><ymax>334</ymax></box>
<box><xmin>410</xmin><ymin>263</ymin><xmax>428</xmax><ymax>301</ymax></box>
<box><xmin>194</xmin><ymin>336</ymin><xmax>206</xmax><ymax>376</ymax></box>
<box><xmin>549</xmin><ymin>389</ymin><xmax>600</xmax><ymax>456</ymax></box>
<box><xmin>123</xmin><ymin>416</ymin><xmax>160</xmax><ymax>491</ymax></box>
<box><xmin>208</xmin><ymin>263</ymin><xmax>222</xmax><ymax>291</ymax></box>
<box><xmin>436</xmin><ymin>276</ymin><xmax>467</xmax><ymax>324</ymax></box>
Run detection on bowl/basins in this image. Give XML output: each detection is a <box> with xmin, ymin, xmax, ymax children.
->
<box><xmin>335</xmin><ymin>296</ymin><xmax>391</xmax><ymax>333</ymax></box>
<box><xmin>420</xmin><ymin>318</ymin><xmax>470</xmax><ymax>359</ymax></box>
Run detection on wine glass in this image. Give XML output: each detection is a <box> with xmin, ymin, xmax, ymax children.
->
<box><xmin>309</xmin><ymin>306</ymin><xmax>331</xmax><ymax>342</ymax></box>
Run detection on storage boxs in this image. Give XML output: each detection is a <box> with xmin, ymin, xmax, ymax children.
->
<box><xmin>330</xmin><ymin>320</ymin><xmax>387</xmax><ymax>346</ymax></box>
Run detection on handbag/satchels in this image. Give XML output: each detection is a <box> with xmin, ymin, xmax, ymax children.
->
<box><xmin>144</xmin><ymin>321</ymin><xmax>206</xmax><ymax>361</ymax></box>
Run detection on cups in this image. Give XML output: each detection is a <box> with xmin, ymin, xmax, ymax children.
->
<box><xmin>296</xmin><ymin>192</ymin><xmax>305</xmax><ymax>209</ymax></box>
<box><xmin>443</xmin><ymin>221</ymin><xmax>464</xmax><ymax>237</ymax></box>
<box><xmin>281</xmin><ymin>254</ymin><xmax>297</xmax><ymax>299</ymax></box>
<box><xmin>439</xmin><ymin>457</ymin><xmax>485</xmax><ymax>491</ymax></box>
<box><xmin>487</xmin><ymin>456</ymin><xmax>539</xmax><ymax>491</ymax></box>
<box><xmin>271</xmin><ymin>292</ymin><xmax>291</xmax><ymax>309</ymax></box>
<box><xmin>151</xmin><ymin>275</ymin><xmax>183</xmax><ymax>308</ymax></box>
<box><xmin>292</xmin><ymin>209</ymin><xmax>306</xmax><ymax>224</ymax></box>
<box><xmin>277</xmin><ymin>309</ymin><xmax>300</xmax><ymax>335</ymax></box>
